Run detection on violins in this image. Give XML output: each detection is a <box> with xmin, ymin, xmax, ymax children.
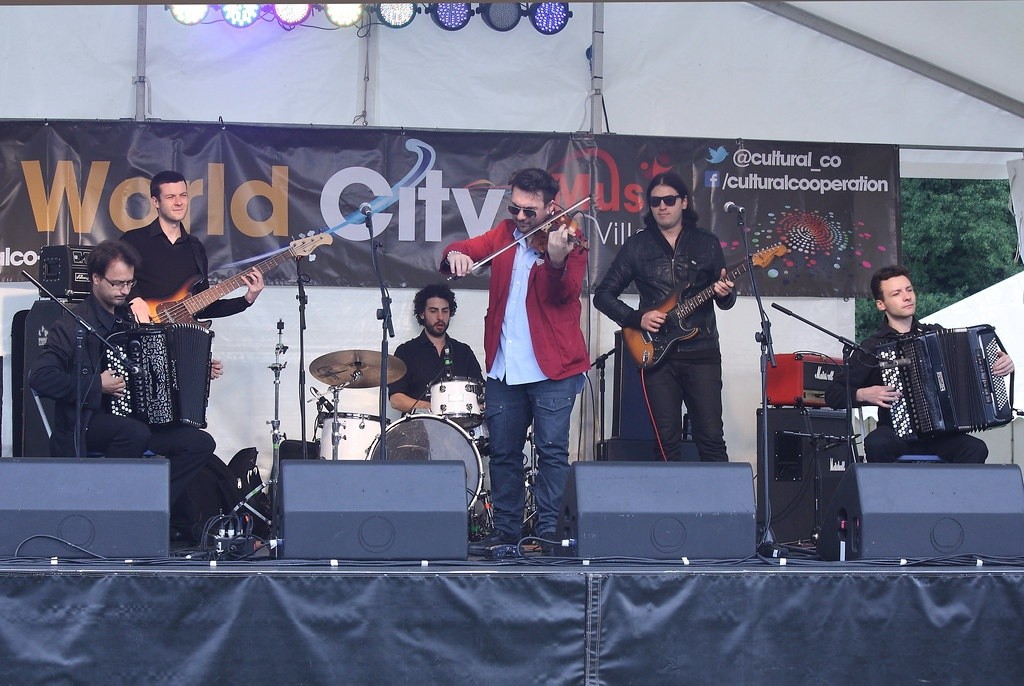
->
<box><xmin>525</xmin><ymin>210</ymin><xmax>591</xmax><ymax>256</ymax></box>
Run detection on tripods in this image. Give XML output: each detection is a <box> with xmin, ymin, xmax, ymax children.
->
<box><xmin>735</xmin><ymin>213</ymin><xmax>817</xmax><ymax>564</ymax></box>
<box><xmin>232</xmin><ymin>322</ymin><xmax>289</xmax><ymax>557</ymax></box>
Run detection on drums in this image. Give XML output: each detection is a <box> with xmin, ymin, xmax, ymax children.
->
<box><xmin>363</xmin><ymin>411</ymin><xmax>485</xmax><ymax>514</ymax></box>
<box><xmin>428</xmin><ymin>375</ymin><xmax>485</xmax><ymax>429</ymax></box>
<box><xmin>317</xmin><ymin>412</ymin><xmax>392</xmax><ymax>461</ymax></box>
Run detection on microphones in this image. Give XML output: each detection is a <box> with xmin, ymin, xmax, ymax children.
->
<box><xmin>313</xmin><ymin>388</ymin><xmax>334</xmax><ymax>411</ymax></box>
<box><xmin>723</xmin><ymin>201</ymin><xmax>746</xmax><ymax>214</ymax></box>
<box><xmin>877</xmin><ymin>358</ymin><xmax>912</xmax><ymax>368</ymax></box>
<box><xmin>360</xmin><ymin>202</ymin><xmax>372</xmax><ymax>216</ymax></box>
<box><xmin>128</xmin><ymin>368</ymin><xmax>150</xmax><ymax>377</ymax></box>
<box><xmin>444</xmin><ymin>346</ymin><xmax>453</xmax><ymax>379</ymax></box>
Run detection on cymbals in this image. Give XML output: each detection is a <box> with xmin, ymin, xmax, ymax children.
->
<box><xmin>309</xmin><ymin>349</ymin><xmax>408</xmax><ymax>389</ymax></box>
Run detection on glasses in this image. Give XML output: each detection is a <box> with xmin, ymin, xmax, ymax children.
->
<box><xmin>506</xmin><ymin>205</ymin><xmax>537</xmax><ymax>220</ymax></box>
<box><xmin>650</xmin><ymin>194</ymin><xmax>681</xmax><ymax>208</ymax></box>
<box><xmin>98</xmin><ymin>272</ymin><xmax>137</xmax><ymax>289</ymax></box>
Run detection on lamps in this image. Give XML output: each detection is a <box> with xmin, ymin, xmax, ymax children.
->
<box><xmin>166</xmin><ymin>2</ymin><xmax>573</xmax><ymax>35</ymax></box>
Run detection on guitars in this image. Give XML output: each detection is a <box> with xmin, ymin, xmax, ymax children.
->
<box><xmin>141</xmin><ymin>228</ymin><xmax>334</xmax><ymax>331</ymax></box>
<box><xmin>622</xmin><ymin>241</ymin><xmax>788</xmax><ymax>375</ymax></box>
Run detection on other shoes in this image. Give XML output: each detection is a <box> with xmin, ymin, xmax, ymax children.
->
<box><xmin>533</xmin><ymin>532</ymin><xmax>560</xmax><ymax>556</ymax></box>
<box><xmin>467</xmin><ymin>528</ymin><xmax>523</xmax><ymax>555</ymax></box>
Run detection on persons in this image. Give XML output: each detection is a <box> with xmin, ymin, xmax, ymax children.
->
<box><xmin>27</xmin><ymin>239</ymin><xmax>223</xmax><ymax>523</ymax></box>
<box><xmin>387</xmin><ymin>283</ymin><xmax>487</xmax><ymax>413</ymax></box>
<box><xmin>592</xmin><ymin>172</ymin><xmax>737</xmax><ymax>461</ymax></box>
<box><xmin>824</xmin><ymin>266</ymin><xmax>1015</xmax><ymax>464</ymax></box>
<box><xmin>439</xmin><ymin>168</ymin><xmax>591</xmax><ymax>557</ymax></box>
<box><xmin>119</xmin><ymin>170</ymin><xmax>264</xmax><ymax>323</ymax></box>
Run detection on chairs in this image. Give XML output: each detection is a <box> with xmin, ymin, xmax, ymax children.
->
<box><xmin>859</xmin><ymin>407</ymin><xmax>942</xmax><ymax>463</ymax></box>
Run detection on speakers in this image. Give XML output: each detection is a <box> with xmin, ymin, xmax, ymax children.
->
<box><xmin>564</xmin><ymin>460</ymin><xmax>760</xmax><ymax>562</ymax></box>
<box><xmin>0</xmin><ymin>457</ymin><xmax>171</xmax><ymax>561</ymax></box>
<box><xmin>813</xmin><ymin>463</ymin><xmax>1024</xmax><ymax>562</ymax></box>
<box><xmin>280</xmin><ymin>458</ymin><xmax>468</xmax><ymax>560</ymax></box>
<box><xmin>758</xmin><ymin>407</ymin><xmax>863</xmax><ymax>559</ymax></box>
<box><xmin>21</xmin><ymin>300</ymin><xmax>81</xmax><ymax>456</ymax></box>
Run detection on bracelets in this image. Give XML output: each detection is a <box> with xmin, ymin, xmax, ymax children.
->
<box><xmin>446</xmin><ymin>250</ymin><xmax>462</xmax><ymax>261</ymax></box>
